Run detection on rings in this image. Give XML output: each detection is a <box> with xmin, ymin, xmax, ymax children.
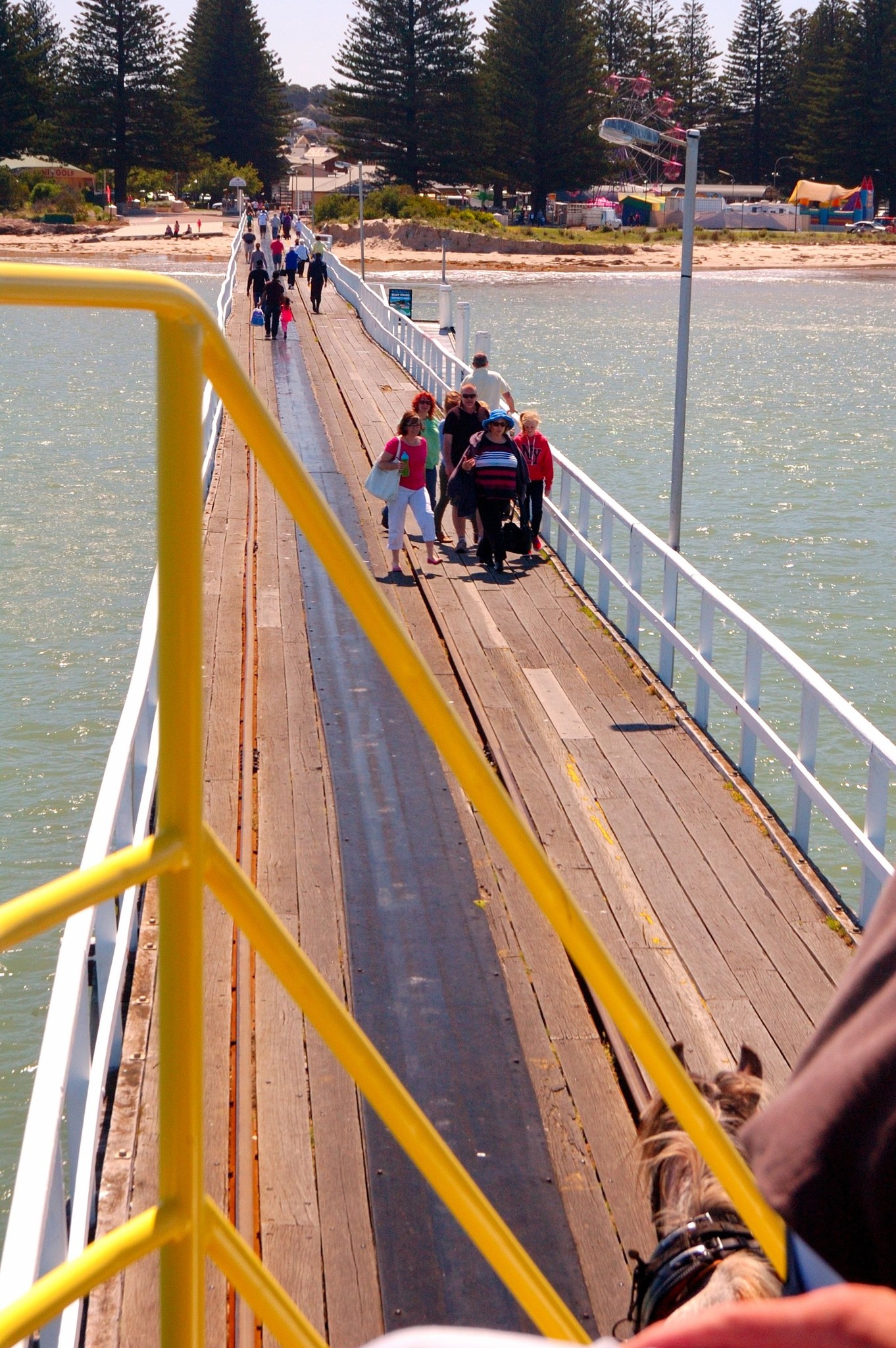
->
<box><xmin>401</xmin><ymin>466</ymin><xmax>402</xmax><ymax>468</ymax></box>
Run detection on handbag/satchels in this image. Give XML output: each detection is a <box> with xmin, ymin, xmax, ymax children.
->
<box><xmin>305</xmin><ymin>255</ymin><xmax>310</xmax><ymax>262</ymax></box>
<box><xmin>251</xmin><ymin>304</ymin><xmax>265</xmax><ymax>326</ymax></box>
<box><xmin>502</xmin><ymin>522</ymin><xmax>533</xmax><ymax>555</ymax></box>
<box><xmin>364</xmin><ymin>437</ymin><xmax>401</xmax><ymax>501</ymax></box>
<box><xmin>279</xmin><ymin>268</ymin><xmax>286</xmax><ymax>277</ymax></box>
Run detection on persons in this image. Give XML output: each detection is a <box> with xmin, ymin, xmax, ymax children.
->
<box><xmin>446</xmin><ymin>409</ymin><xmax>531</xmax><ymax>571</ymax></box>
<box><xmin>362</xmin><ymin>1283</ymin><xmax>896</xmax><ymax>1348</ymax></box>
<box><xmin>165</xmin><ymin>219</ymin><xmax>202</xmax><ymax>237</ymax></box>
<box><xmin>627</xmin><ymin>212</ymin><xmax>641</xmax><ymax>227</ymax></box>
<box><xmin>257</xmin><ymin>271</ymin><xmax>286</xmax><ymax>342</ymax></box>
<box><xmin>518</xmin><ymin>208</ymin><xmax>544</xmax><ymax>228</ymax></box>
<box><xmin>242</xmin><ymin>200</ymin><xmax>328</xmax><ymax>315</ymax></box>
<box><xmin>278</xmin><ymin>298</ymin><xmax>295</xmax><ymax>340</ymax></box>
<box><xmin>378</xmin><ymin>411</ymin><xmax>442</xmax><ymax>571</ymax></box>
<box><xmin>127</xmin><ymin>194</ymin><xmax>133</xmax><ymax>201</ymax></box>
<box><xmin>335</xmin><ymin>173</ymin><xmax>336</xmax><ymax>178</ymax></box>
<box><xmin>408</xmin><ymin>352</ymin><xmax>553</xmax><ymax>554</ymax></box>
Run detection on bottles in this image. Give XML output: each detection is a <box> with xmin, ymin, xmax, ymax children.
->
<box><xmin>401</xmin><ymin>451</ymin><xmax>410</xmax><ymax>477</ymax></box>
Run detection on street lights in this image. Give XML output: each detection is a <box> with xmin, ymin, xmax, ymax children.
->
<box><xmin>641</xmin><ymin>176</ymin><xmax>648</xmax><ymax>223</ymax></box>
<box><xmin>773</xmin><ymin>156</ymin><xmax>795</xmax><ymax>191</ymax></box>
<box><xmin>592</xmin><ymin>117</ymin><xmax>700</xmax><ymax>550</ymax></box>
<box><xmin>718</xmin><ymin>169</ymin><xmax>736</xmax><ymax>204</ymax></box>
<box><xmin>659</xmin><ymin>183</ymin><xmax>663</xmax><ymax>198</ymax></box>
<box><xmin>335</xmin><ymin>159</ymin><xmax>365</xmax><ymax>282</ymax></box>
<box><xmin>740</xmin><ymin>198</ymin><xmax>749</xmax><ymax>236</ymax></box>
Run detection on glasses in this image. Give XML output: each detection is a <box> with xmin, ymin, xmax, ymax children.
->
<box><xmin>487</xmin><ymin>421</ymin><xmax>509</xmax><ymax>428</ymax></box>
<box><xmin>406</xmin><ymin>423</ymin><xmax>421</xmax><ymax>428</ymax></box>
<box><xmin>419</xmin><ymin>400</ymin><xmax>432</xmax><ymax>406</ymax></box>
<box><xmin>462</xmin><ymin>393</ymin><xmax>477</xmax><ymax>398</ymax></box>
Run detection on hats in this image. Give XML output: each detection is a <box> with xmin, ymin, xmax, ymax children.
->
<box><xmin>482</xmin><ymin>409</ymin><xmax>514</xmax><ymax>431</ymax></box>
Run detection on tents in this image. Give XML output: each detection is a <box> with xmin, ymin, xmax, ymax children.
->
<box><xmin>0</xmin><ymin>147</ymin><xmax>96</xmax><ymax>193</ymax></box>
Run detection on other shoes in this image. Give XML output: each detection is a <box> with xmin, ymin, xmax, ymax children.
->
<box><xmin>265</xmin><ymin>333</ymin><xmax>271</xmax><ymax>337</ymax></box>
<box><xmin>382</xmin><ymin>508</ymin><xmax>390</xmax><ymax>529</ymax></box>
<box><xmin>428</xmin><ymin>558</ymin><xmax>443</xmax><ymax>564</ymax></box>
<box><xmin>524</xmin><ymin>549</ymin><xmax>532</xmax><ymax>557</ymax></box>
<box><xmin>434</xmin><ymin>531</ymin><xmax>453</xmax><ymax>542</ymax></box>
<box><xmin>532</xmin><ymin>536</ymin><xmax>541</xmax><ymax>551</ymax></box>
<box><xmin>478</xmin><ymin>557</ymin><xmax>496</xmax><ymax>566</ymax></box>
<box><xmin>392</xmin><ymin>564</ymin><xmax>402</xmax><ymax>571</ymax></box>
<box><xmin>494</xmin><ymin>560</ymin><xmax>504</xmax><ymax>571</ymax></box>
<box><xmin>265</xmin><ymin>274</ymin><xmax>303</xmax><ymax>290</ymax></box>
<box><xmin>284</xmin><ymin>332</ymin><xmax>287</xmax><ymax>339</ymax></box>
<box><xmin>312</xmin><ymin>306</ymin><xmax>320</xmax><ymax>314</ymax></box>
<box><xmin>272</xmin><ymin>336</ymin><xmax>276</xmax><ymax>340</ymax></box>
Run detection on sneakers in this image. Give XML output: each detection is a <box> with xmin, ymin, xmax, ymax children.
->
<box><xmin>456</xmin><ymin>541</ymin><xmax>466</xmax><ymax>552</ymax></box>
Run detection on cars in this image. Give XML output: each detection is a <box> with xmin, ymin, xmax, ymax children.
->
<box><xmin>212</xmin><ymin>199</ymin><xmax>247</xmax><ymax>211</ymax></box>
<box><xmin>845</xmin><ymin>217</ymin><xmax>896</xmax><ymax>236</ymax></box>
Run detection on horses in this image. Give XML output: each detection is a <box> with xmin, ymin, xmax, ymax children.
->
<box><xmin>632</xmin><ymin>1042</ymin><xmax>783</xmax><ymax>1332</ymax></box>
